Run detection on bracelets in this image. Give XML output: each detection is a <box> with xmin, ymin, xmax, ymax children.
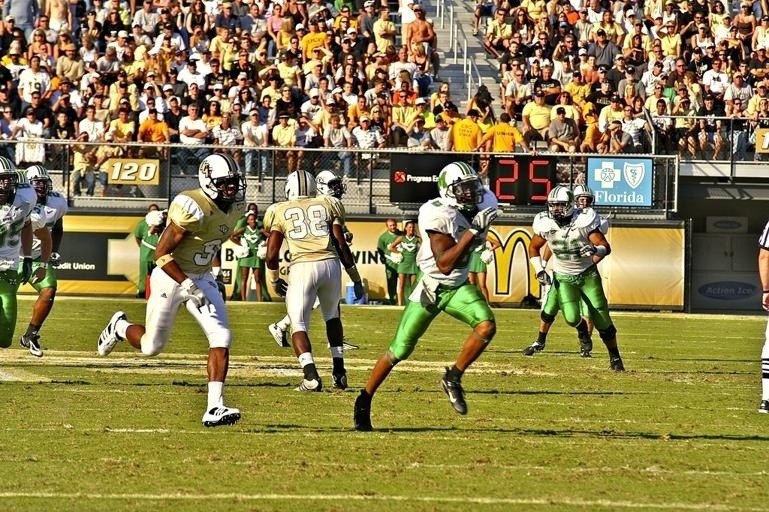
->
<box><xmin>40</xmin><ymin>262</ymin><xmax>49</xmax><ymax>270</ymax></box>
<box><xmin>763</xmin><ymin>290</ymin><xmax>769</xmax><ymax>294</ymax></box>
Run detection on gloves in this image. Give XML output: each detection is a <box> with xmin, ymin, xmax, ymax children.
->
<box><xmin>471</xmin><ymin>207</ymin><xmax>497</xmax><ymax>231</ymax></box>
<box><xmin>271</xmin><ymin>278</ymin><xmax>288</xmax><ymax>297</ymax></box>
<box><xmin>213</xmin><ymin>274</ymin><xmax>226</xmax><ymax>301</ymax></box>
<box><xmin>19</xmin><ymin>258</ymin><xmax>33</xmax><ymax>284</ymax></box>
<box><xmin>579</xmin><ymin>244</ymin><xmax>595</xmax><ymax>257</ymax></box>
<box><xmin>536</xmin><ymin>270</ymin><xmax>551</xmax><ymax>285</ymax></box>
<box><xmin>50</xmin><ymin>251</ymin><xmax>64</xmax><ymax>266</ymax></box>
<box><xmin>354</xmin><ymin>281</ymin><xmax>363</xmax><ymax>301</ymax></box>
<box><xmin>187</xmin><ymin>284</ymin><xmax>212</xmax><ymax>312</ymax></box>
<box><xmin>761</xmin><ymin>292</ymin><xmax>769</xmax><ymax>311</ymax></box>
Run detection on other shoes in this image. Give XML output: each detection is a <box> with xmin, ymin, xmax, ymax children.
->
<box><xmin>473</xmin><ymin>29</ymin><xmax>479</xmax><ymax>36</ymax></box>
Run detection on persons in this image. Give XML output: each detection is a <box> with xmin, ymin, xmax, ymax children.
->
<box><xmin>263</xmin><ymin>170</ymin><xmax>364</xmax><ymax>392</ymax></box>
<box><xmin>1</xmin><ymin>155</ymin><xmax>495</xmax><ymax>353</ymax></box>
<box><xmin>521</xmin><ymin>186</ymin><xmax>625</xmax><ymax>373</ymax></box>
<box><xmin>757</xmin><ymin>216</ymin><xmax>769</xmax><ymax>415</ymax></box>
<box><xmin>97</xmin><ymin>152</ymin><xmax>247</xmax><ymax>427</ymax></box>
<box><xmin>1</xmin><ymin>0</ymin><xmax>768</xmax><ymax>197</ymax></box>
<box><xmin>353</xmin><ymin>160</ymin><xmax>505</xmax><ymax>431</ymax></box>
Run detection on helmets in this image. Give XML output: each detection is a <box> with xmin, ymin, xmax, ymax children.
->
<box><xmin>574</xmin><ymin>185</ymin><xmax>595</xmax><ymax>207</ymax></box>
<box><xmin>0</xmin><ymin>156</ymin><xmax>19</xmax><ymax>196</ymax></box>
<box><xmin>316</xmin><ymin>170</ymin><xmax>346</xmax><ymax>199</ymax></box>
<box><xmin>547</xmin><ymin>186</ymin><xmax>573</xmax><ymax>219</ymax></box>
<box><xmin>438</xmin><ymin>161</ymin><xmax>483</xmax><ymax>210</ymax></box>
<box><xmin>199</xmin><ymin>153</ymin><xmax>246</xmax><ymax>202</ymax></box>
<box><xmin>285</xmin><ymin>170</ymin><xmax>317</xmax><ymax>200</ymax></box>
<box><xmin>24</xmin><ymin>165</ymin><xmax>52</xmax><ymax>198</ymax></box>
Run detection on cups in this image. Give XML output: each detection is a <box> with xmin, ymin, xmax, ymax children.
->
<box><xmin>373</xmin><ymin>301</ymin><xmax>378</xmax><ymax>305</ymax></box>
<box><xmin>369</xmin><ymin>301</ymin><xmax>372</xmax><ymax>305</ymax></box>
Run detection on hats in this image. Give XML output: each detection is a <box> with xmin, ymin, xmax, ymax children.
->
<box><xmin>0</xmin><ymin>0</ymin><xmax>453</xmax><ymax>123</ymax></box>
<box><xmin>497</xmin><ymin>1</ymin><xmax>759</xmax><ymax>122</ymax></box>
<box><xmin>466</xmin><ymin>85</ymin><xmax>495</xmax><ymax>118</ymax></box>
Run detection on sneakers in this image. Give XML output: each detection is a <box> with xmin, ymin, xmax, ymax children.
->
<box><xmin>354</xmin><ymin>393</ymin><xmax>372</xmax><ymax>431</ymax></box>
<box><xmin>202</xmin><ymin>406</ymin><xmax>240</xmax><ymax>426</ymax></box>
<box><xmin>441</xmin><ymin>366</ymin><xmax>466</xmax><ymax>414</ymax></box>
<box><xmin>758</xmin><ymin>400</ymin><xmax>769</xmax><ymax>414</ymax></box>
<box><xmin>332</xmin><ymin>371</ymin><xmax>347</xmax><ymax>389</ymax></box>
<box><xmin>294</xmin><ymin>378</ymin><xmax>321</xmax><ymax>391</ymax></box>
<box><xmin>97</xmin><ymin>311</ymin><xmax>126</xmax><ymax>356</ymax></box>
<box><xmin>269</xmin><ymin>322</ymin><xmax>290</xmax><ymax>346</ymax></box>
<box><xmin>522</xmin><ymin>330</ymin><xmax>624</xmax><ymax>370</ymax></box>
<box><xmin>20</xmin><ymin>335</ymin><xmax>43</xmax><ymax>357</ymax></box>
